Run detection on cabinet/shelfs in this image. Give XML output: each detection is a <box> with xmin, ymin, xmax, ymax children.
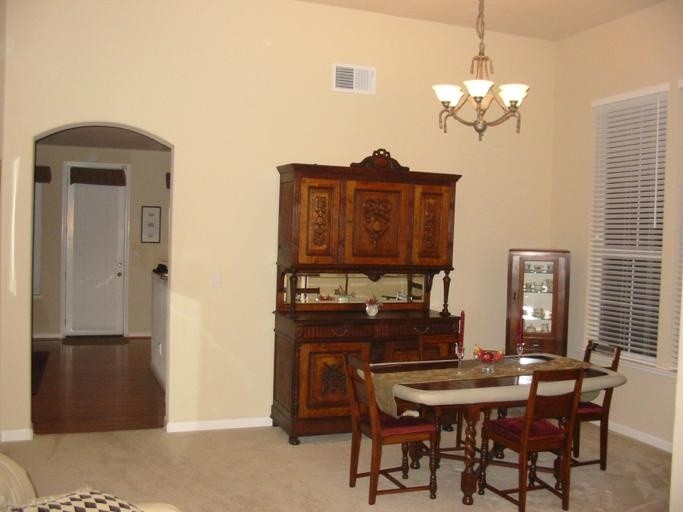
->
<box><xmin>268</xmin><ymin>148</ymin><xmax>464</xmax><ymax>446</ymax></box>
<box><xmin>501</xmin><ymin>246</ymin><xmax>573</xmax><ymax>358</ymax></box>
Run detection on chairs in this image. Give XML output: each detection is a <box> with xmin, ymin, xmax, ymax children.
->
<box><xmin>478</xmin><ymin>366</ymin><xmax>584</xmax><ymax>510</ymax></box>
<box><xmin>337</xmin><ymin>341</ymin><xmax>440</xmax><ymax>506</ymax></box>
<box><xmin>558</xmin><ymin>338</ymin><xmax>622</xmax><ymax>472</ymax></box>
<box><xmin>417</xmin><ymin>333</ymin><xmax>465</xmax><ymax>450</ymax></box>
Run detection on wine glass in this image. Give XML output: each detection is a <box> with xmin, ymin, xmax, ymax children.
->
<box><xmin>515</xmin><ymin>343</ymin><xmax>523</xmax><ymax>364</ymax></box>
<box><xmin>455</xmin><ymin>348</ymin><xmax>466</xmax><ymax>373</ymax></box>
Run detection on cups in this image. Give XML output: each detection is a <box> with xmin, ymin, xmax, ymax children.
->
<box><xmin>473</xmin><ymin>347</ymin><xmax>502</xmax><ymax>374</ymax></box>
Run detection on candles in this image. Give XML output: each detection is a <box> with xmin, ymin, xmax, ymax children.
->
<box><xmin>459</xmin><ymin>310</ymin><xmax>463</xmax><ymax>336</ymax></box>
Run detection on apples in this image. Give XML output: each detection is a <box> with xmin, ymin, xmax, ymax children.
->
<box><xmin>481</xmin><ymin>352</ymin><xmax>494</xmax><ymax>363</ymax></box>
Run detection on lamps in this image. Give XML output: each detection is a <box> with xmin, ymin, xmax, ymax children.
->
<box><xmin>427</xmin><ymin>1</ymin><xmax>528</xmax><ymax>147</ymax></box>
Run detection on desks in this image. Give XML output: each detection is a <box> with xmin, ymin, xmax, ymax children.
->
<box><xmin>356</xmin><ymin>351</ymin><xmax>627</xmax><ymax>506</ymax></box>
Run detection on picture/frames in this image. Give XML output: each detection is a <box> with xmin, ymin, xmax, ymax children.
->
<box><xmin>139</xmin><ymin>205</ymin><xmax>161</xmax><ymax>244</ymax></box>
